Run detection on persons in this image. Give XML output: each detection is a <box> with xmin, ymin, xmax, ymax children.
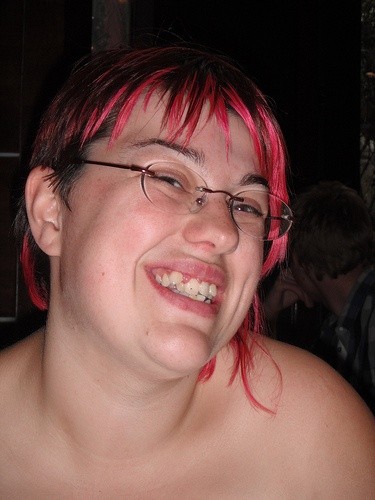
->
<box><xmin>256</xmin><ymin>180</ymin><xmax>374</xmax><ymax>415</ymax></box>
<box><xmin>0</xmin><ymin>44</ymin><xmax>372</xmax><ymax>500</ymax></box>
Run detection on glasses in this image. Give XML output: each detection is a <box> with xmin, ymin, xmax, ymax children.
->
<box><xmin>71</xmin><ymin>156</ymin><xmax>293</xmax><ymax>240</ymax></box>
